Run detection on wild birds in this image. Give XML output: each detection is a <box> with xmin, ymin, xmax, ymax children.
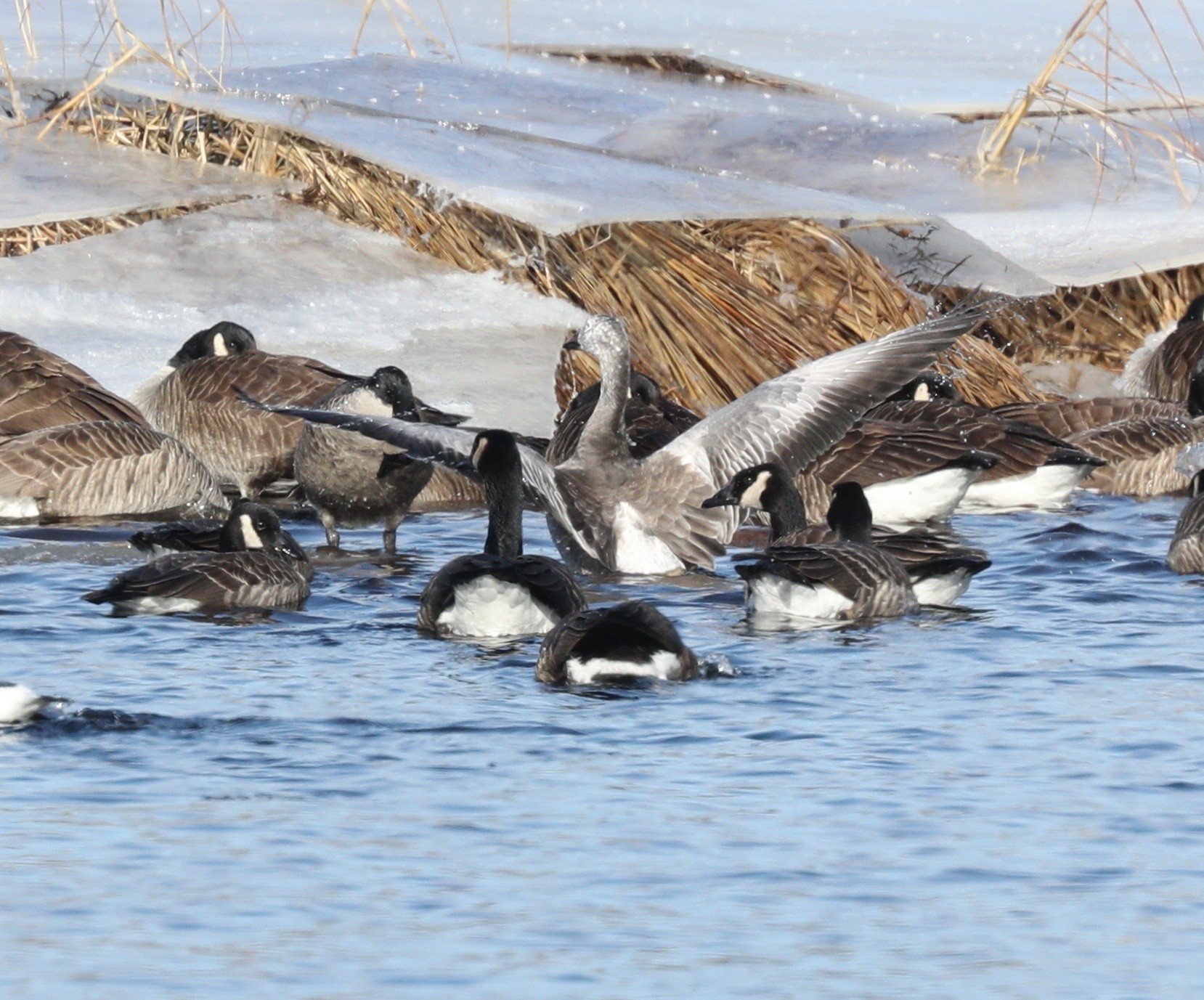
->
<box><xmin>534</xmin><ymin>598</ymin><xmax>718</xmax><ymax>695</ymax></box>
<box><xmin>988</xmin><ymin>397</ymin><xmax>1189</xmax><ymax>440</ymax></box>
<box><xmin>1058</xmin><ymin>414</ymin><xmax>1204</xmax><ymax>498</ymax></box>
<box><xmin>0</xmin><ymin>420</ymin><xmax>341</xmax><ymax>529</ymax></box>
<box><xmin>820</xmin><ymin>482</ymin><xmax>992</xmax><ymax>607</ymax></box>
<box><xmin>125</xmin><ymin>320</ymin><xmax>471</xmax><ymax>496</ymax></box>
<box><xmin>701</xmin><ymin>464</ymin><xmax>921</xmax><ymax>630</ymax></box>
<box><xmin>418</xmin><ymin>430</ymin><xmax>588</xmax><ymax>654</ymax></box>
<box><xmin>786</xmin><ymin>417</ymin><xmax>1000</xmax><ymax>541</ymax></box>
<box><xmin>227</xmin><ymin>292</ymin><xmax>1011</xmax><ymax>589</ymax></box>
<box><xmin>849</xmin><ymin>369</ymin><xmax>1106</xmax><ymax>513</ymax></box>
<box><xmin>80</xmin><ymin>502</ymin><xmax>310</xmax><ymax>628</ymax></box>
<box><xmin>292</xmin><ymin>366</ymin><xmax>433</xmax><ymax>560</ymax></box>
<box><xmin>0</xmin><ymin>329</ymin><xmax>151</xmax><ymax>443</ymax></box>
<box><xmin>1120</xmin><ymin>292</ymin><xmax>1204</xmax><ymax>418</ymax></box>
<box><xmin>1168</xmin><ymin>467</ymin><xmax>1204</xmax><ymax>575</ymax></box>
<box><xmin>544</xmin><ymin>373</ymin><xmax>707</xmax><ymax>466</ymax></box>
